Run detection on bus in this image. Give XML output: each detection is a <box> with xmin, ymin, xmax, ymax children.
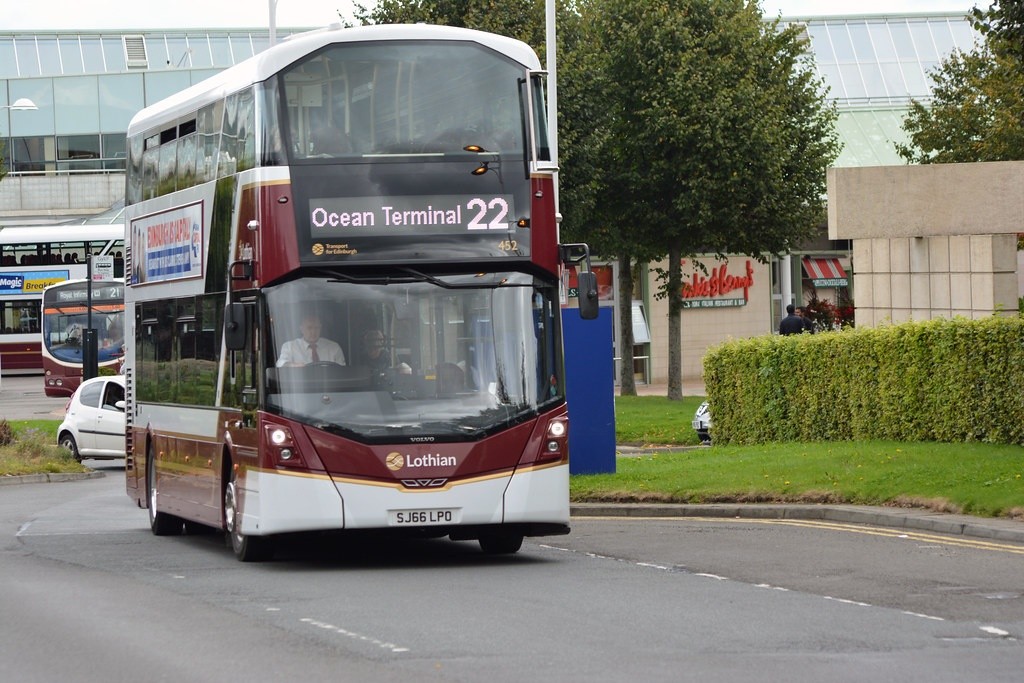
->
<box><xmin>40</xmin><ymin>277</ymin><xmax>125</xmax><ymax>398</ymax></box>
<box><xmin>0</xmin><ymin>225</ymin><xmax>125</xmax><ymax>377</ymax></box>
<box><xmin>123</xmin><ymin>24</ymin><xmax>599</xmax><ymax>565</ymax></box>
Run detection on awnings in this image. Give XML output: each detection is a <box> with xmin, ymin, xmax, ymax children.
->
<box><xmin>803</xmin><ymin>258</ymin><xmax>847</xmax><ymax>286</ymax></box>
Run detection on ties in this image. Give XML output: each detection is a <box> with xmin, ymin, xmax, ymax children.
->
<box><xmin>310</xmin><ymin>344</ymin><xmax>320</xmax><ymax>362</ymax></box>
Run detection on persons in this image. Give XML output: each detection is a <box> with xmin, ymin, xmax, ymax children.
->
<box><xmin>276</xmin><ymin>311</ymin><xmax>412</xmax><ymax>372</ymax></box>
<box><xmin>777</xmin><ymin>305</ymin><xmax>813</xmax><ymax>335</ymax></box>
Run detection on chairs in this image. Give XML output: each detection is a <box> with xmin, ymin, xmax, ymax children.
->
<box><xmin>3</xmin><ymin>251</ymin><xmax>124</xmax><ymax>278</ymax></box>
<box><xmin>5</xmin><ymin>327</ymin><xmax>41</xmax><ymax>334</ymax></box>
<box><xmin>158</xmin><ymin>331</ymin><xmax>466</xmax><ymax>400</ymax></box>
<box><xmin>312</xmin><ymin>128</ymin><xmax>500</xmax><ymax>155</ymax></box>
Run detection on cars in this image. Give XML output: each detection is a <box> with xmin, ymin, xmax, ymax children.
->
<box><xmin>57</xmin><ymin>375</ymin><xmax>126</xmax><ymax>465</ymax></box>
